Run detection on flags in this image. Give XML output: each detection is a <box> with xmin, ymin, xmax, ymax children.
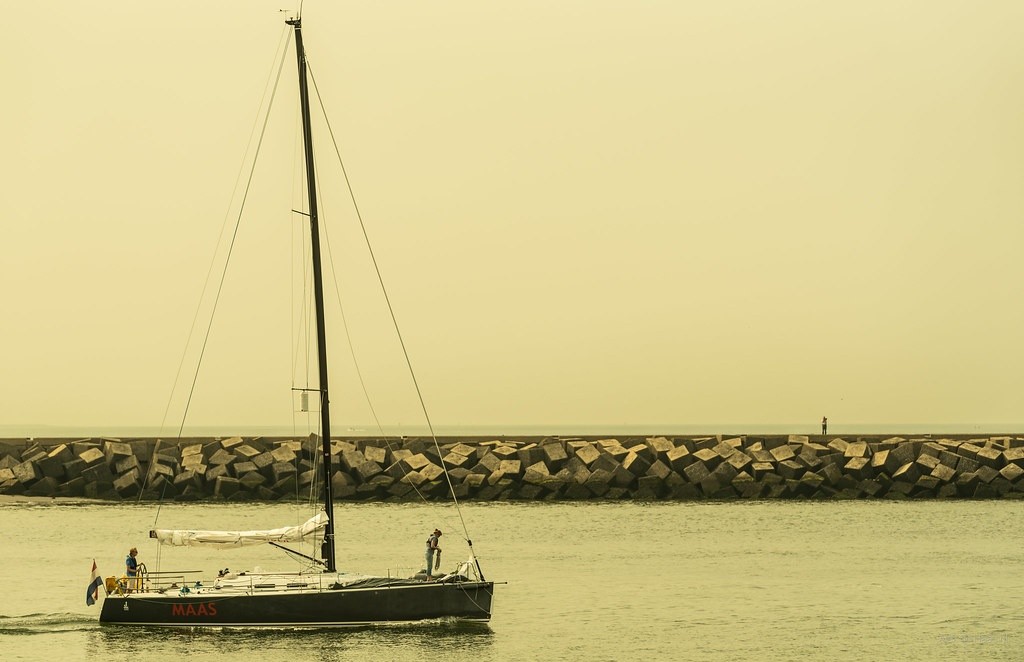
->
<box><xmin>86</xmin><ymin>562</ymin><xmax>103</xmax><ymax>606</ymax></box>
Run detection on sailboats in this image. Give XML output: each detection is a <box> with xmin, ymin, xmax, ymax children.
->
<box><xmin>97</xmin><ymin>0</ymin><xmax>494</xmax><ymax>631</ymax></box>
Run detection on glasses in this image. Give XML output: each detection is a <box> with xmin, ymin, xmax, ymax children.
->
<box><xmin>133</xmin><ymin>551</ymin><xmax>137</xmax><ymax>553</ymax></box>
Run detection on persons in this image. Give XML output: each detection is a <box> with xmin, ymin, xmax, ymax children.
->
<box><xmin>126</xmin><ymin>548</ymin><xmax>138</xmax><ymax>594</ymax></box>
<box><xmin>821</xmin><ymin>416</ymin><xmax>827</xmax><ymax>435</ymax></box>
<box><xmin>425</xmin><ymin>528</ymin><xmax>442</xmax><ymax>581</ymax></box>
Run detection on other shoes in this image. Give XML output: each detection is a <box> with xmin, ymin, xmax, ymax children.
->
<box><xmin>426</xmin><ymin>579</ymin><xmax>435</xmax><ymax>583</ymax></box>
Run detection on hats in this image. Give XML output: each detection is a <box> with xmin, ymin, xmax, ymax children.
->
<box><xmin>824</xmin><ymin>417</ymin><xmax>827</xmax><ymax>421</ymax></box>
